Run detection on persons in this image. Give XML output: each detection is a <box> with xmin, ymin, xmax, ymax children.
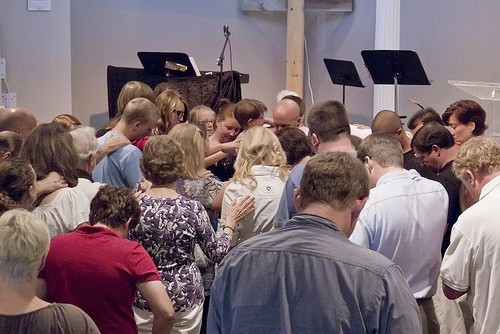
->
<box><xmin>0</xmin><ymin>79</ymin><xmax>500</xmax><ymax>334</ymax></box>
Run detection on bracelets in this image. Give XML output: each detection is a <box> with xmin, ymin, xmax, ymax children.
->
<box><xmin>223</xmin><ymin>226</ymin><xmax>235</xmax><ymax>234</ymax></box>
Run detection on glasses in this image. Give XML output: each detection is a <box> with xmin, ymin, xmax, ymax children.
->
<box><xmin>174</xmin><ymin>110</ymin><xmax>185</xmax><ymax>119</ymax></box>
<box><xmin>198</xmin><ymin>121</ymin><xmax>216</xmax><ymax>125</ymax></box>
